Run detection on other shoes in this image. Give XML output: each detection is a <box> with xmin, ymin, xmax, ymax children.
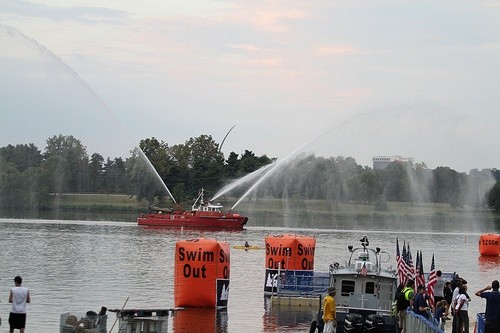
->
<box><xmin>445</xmin><ymin>316</ymin><xmax>451</xmax><ymax>320</ymax></box>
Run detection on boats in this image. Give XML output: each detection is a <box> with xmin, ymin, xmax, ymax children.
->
<box><xmin>309</xmin><ymin>235</ymin><xmax>402</xmax><ymax>333</ymax></box>
<box><xmin>137</xmin><ymin>186</ymin><xmax>249</xmax><ymax>230</ymax></box>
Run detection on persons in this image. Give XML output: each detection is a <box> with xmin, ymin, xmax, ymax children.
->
<box><xmin>9</xmin><ymin>276</ymin><xmax>30</xmax><ymax>333</ymax></box>
<box><xmin>173</xmin><ymin>209</ymin><xmax>191</xmax><ymax>218</ymax></box>
<box><xmin>245</xmin><ymin>242</ymin><xmax>248</xmax><ymax>246</ymax></box>
<box><xmin>475</xmin><ymin>280</ymin><xmax>500</xmax><ymax>333</ymax></box>
<box><xmin>323</xmin><ymin>287</ymin><xmax>337</xmax><ymax>333</ymax></box>
<box><xmin>394</xmin><ymin>270</ymin><xmax>471</xmax><ymax>333</ymax></box>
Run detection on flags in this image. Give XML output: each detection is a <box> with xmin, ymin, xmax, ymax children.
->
<box><xmin>397</xmin><ymin>242</ymin><xmax>437</xmax><ymax>309</ymax></box>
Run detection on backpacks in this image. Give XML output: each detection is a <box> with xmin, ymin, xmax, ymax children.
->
<box><xmin>395</xmin><ymin>288</ymin><xmax>412</xmax><ymax>311</ymax></box>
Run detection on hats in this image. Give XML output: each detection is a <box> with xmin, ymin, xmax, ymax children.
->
<box><xmin>327</xmin><ymin>287</ymin><xmax>334</xmax><ymax>294</ymax></box>
<box><xmin>418</xmin><ymin>284</ymin><xmax>425</xmax><ymax>289</ymax></box>
